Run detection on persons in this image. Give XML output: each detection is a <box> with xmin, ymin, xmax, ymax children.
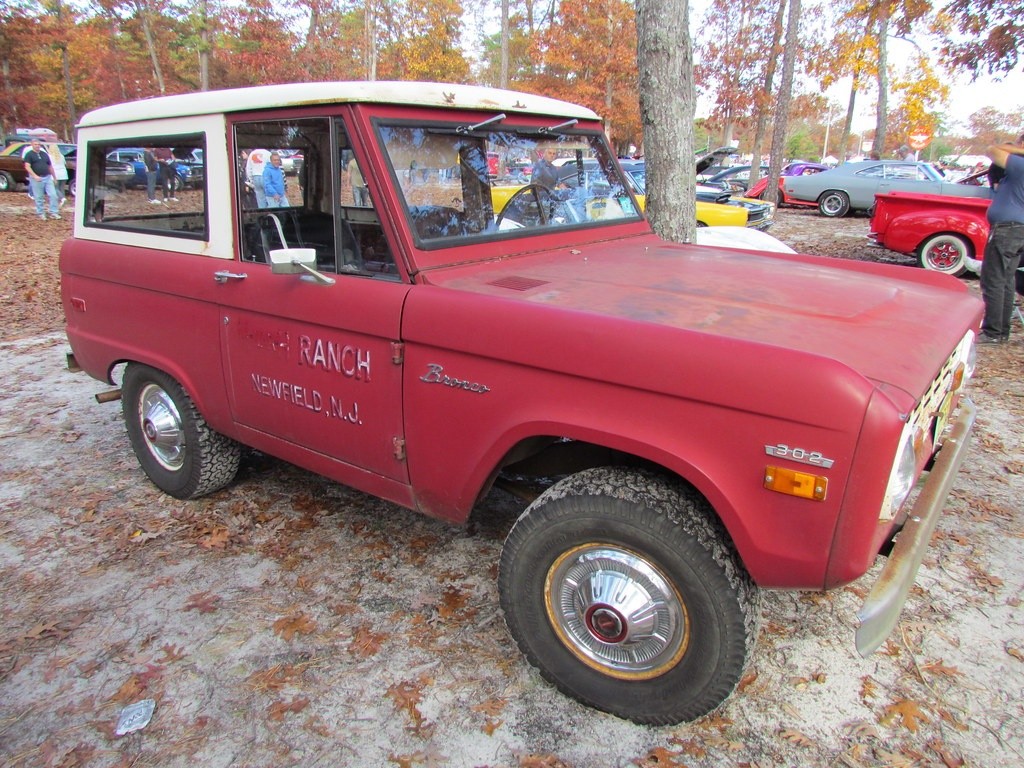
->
<box><xmin>234</xmin><ymin>147</ymin><xmax>309</xmax><ymax>211</ymax></box>
<box><xmin>529</xmin><ymin>147</ymin><xmax>558</xmax><ymax>229</ymax></box>
<box><xmin>974</xmin><ymin>133</ymin><xmax>1024</xmax><ymax>345</ymax></box>
<box><xmin>22</xmin><ymin>136</ymin><xmax>69</xmax><ymax>222</ymax></box>
<box><xmin>153</xmin><ymin>146</ymin><xmax>179</xmax><ymax>202</ymax></box>
<box><xmin>346</xmin><ymin>156</ymin><xmax>369</xmax><ymax>206</ymax></box>
<box><xmin>143</xmin><ymin>146</ymin><xmax>162</xmax><ymax>205</ymax></box>
<box><xmin>513</xmin><ymin>136</ymin><xmax>999</xmax><ymax>202</ymax></box>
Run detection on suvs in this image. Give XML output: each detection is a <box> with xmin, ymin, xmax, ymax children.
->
<box><xmin>58</xmin><ymin>81</ymin><xmax>986</xmax><ymax>728</ymax></box>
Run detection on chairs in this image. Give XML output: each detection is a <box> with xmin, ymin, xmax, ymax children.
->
<box><xmin>408</xmin><ymin>205</ymin><xmax>471</xmax><ymax>242</ymax></box>
<box><xmin>255</xmin><ymin>207</ymin><xmax>363</xmax><ymax>273</ymax></box>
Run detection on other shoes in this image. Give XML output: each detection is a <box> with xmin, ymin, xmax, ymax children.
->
<box><xmin>170</xmin><ymin>198</ymin><xmax>179</xmax><ymax>201</ymax></box>
<box><xmin>50</xmin><ymin>214</ymin><xmax>62</xmax><ymax>220</ymax></box>
<box><xmin>164</xmin><ymin>198</ymin><xmax>168</xmax><ymax>202</ymax></box>
<box><xmin>148</xmin><ymin>198</ymin><xmax>162</xmax><ymax>204</ymax></box>
<box><xmin>60</xmin><ymin>197</ymin><xmax>66</xmax><ymax>208</ymax></box>
<box><xmin>977</xmin><ymin>331</ymin><xmax>1009</xmax><ymax>343</ymax></box>
<box><xmin>39</xmin><ymin>215</ymin><xmax>48</xmax><ymax>222</ymax></box>
<box><xmin>28</xmin><ymin>193</ymin><xmax>35</xmax><ymax>199</ymax></box>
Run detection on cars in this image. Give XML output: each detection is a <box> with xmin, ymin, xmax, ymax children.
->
<box><xmin>0</xmin><ymin>133</ymin><xmax>305</xmax><ymax>198</ymax></box>
<box><xmin>538</xmin><ymin>144</ymin><xmax>1012</xmax><ymax>234</ymax></box>
